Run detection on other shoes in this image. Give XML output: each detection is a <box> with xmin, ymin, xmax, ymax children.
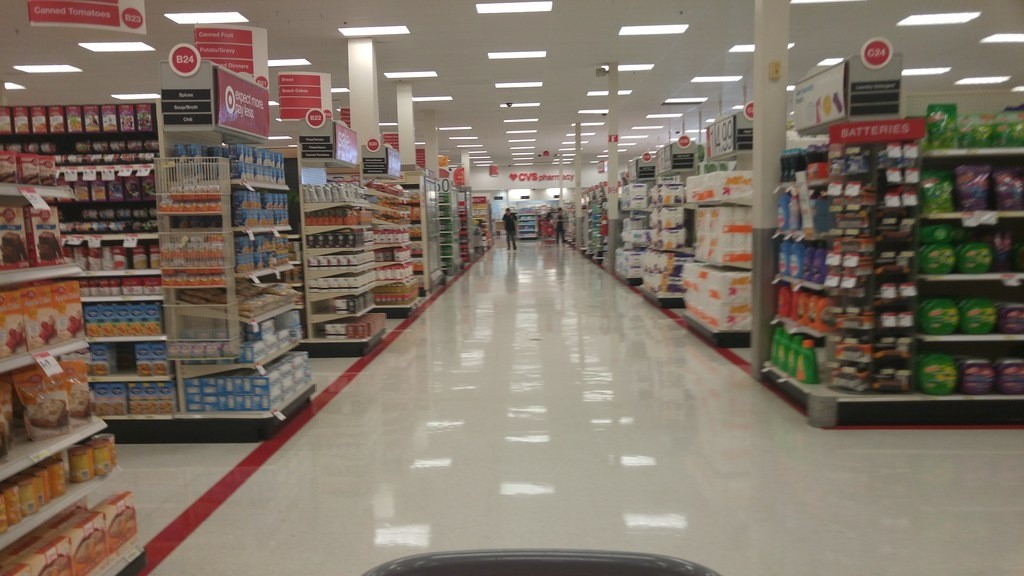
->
<box><xmin>562</xmin><ymin>240</ymin><xmax>567</xmax><ymax>243</ymax></box>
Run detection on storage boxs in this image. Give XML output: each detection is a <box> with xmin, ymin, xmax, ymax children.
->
<box><xmin>85</xmin><ymin>305</ymin><xmax>310</xmax><ymax>416</ymax></box>
<box><xmin>1</xmin><ymin>491</ymin><xmax>139</xmax><ymax>576</ymax></box>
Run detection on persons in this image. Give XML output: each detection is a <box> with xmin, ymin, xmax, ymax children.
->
<box><xmin>502</xmin><ymin>208</ymin><xmax>517</xmax><ymax>250</ymax></box>
<box><xmin>555</xmin><ymin>207</ymin><xmax>567</xmax><ymax>244</ymax></box>
<box><xmin>546</xmin><ymin>209</ymin><xmax>554</xmax><ymax>221</ymax></box>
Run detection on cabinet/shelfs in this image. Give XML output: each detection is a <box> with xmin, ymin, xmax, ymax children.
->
<box><xmin>515</xmin><ymin>213</ymin><xmax>538</xmax><ymax>238</ymax></box>
<box><xmin>583</xmin><ymin>140</ymin><xmax>1024</xmax><ymax>428</ymax></box>
<box><xmin>292</xmin><ymin>164</ymin><xmax>492</xmax><ymax>361</ymax></box>
<box><xmin>0</xmin><ymin>183</ymin><xmax>147</xmax><ymax>576</ymax></box>
<box><xmin>0</xmin><ymin>103</ymin><xmax>317</xmax><ymax>444</ymax></box>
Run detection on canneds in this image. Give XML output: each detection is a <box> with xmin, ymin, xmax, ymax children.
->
<box><xmin>173</xmin><ymin>143</ymin><xmax>289</xmax><ymax>273</ymax></box>
<box><xmin>63</xmin><ymin>243</ymin><xmax>162</xmax><ymax>296</ymax></box>
<box><xmin>0</xmin><ymin>432</ymin><xmax>117</xmax><ymax>534</ymax></box>
